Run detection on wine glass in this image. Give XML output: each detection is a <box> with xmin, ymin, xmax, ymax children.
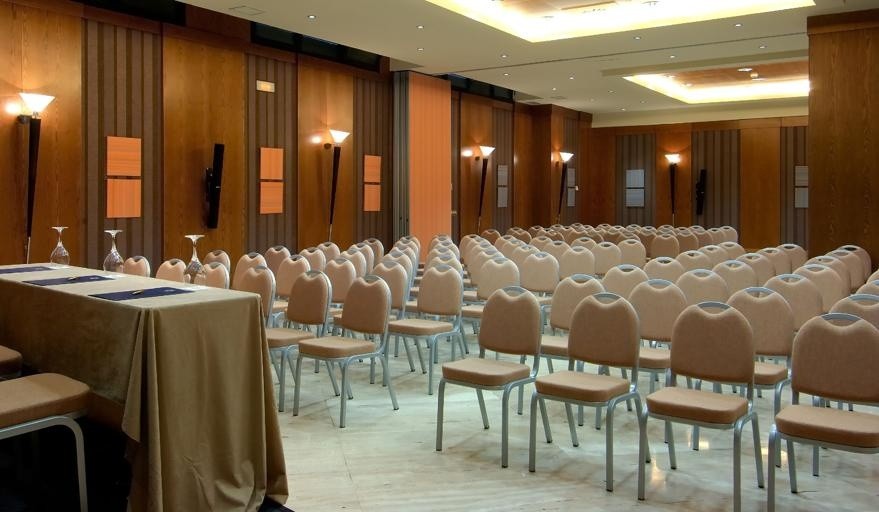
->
<box><xmin>182</xmin><ymin>233</ymin><xmax>208</xmax><ymax>289</ymax></box>
<box><xmin>49</xmin><ymin>226</ymin><xmax>70</xmax><ymax>268</ymax></box>
<box><xmin>102</xmin><ymin>229</ymin><xmax>126</xmax><ymax>275</ymax></box>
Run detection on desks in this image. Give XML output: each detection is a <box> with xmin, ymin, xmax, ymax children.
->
<box><xmin>0</xmin><ymin>264</ymin><xmax>289</xmax><ymax>511</ymax></box>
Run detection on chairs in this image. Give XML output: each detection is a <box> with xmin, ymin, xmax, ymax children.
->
<box><xmin>381</xmin><ymin>263</ymin><xmax>466</xmax><ymax>397</ymax></box>
<box><xmin>434</xmin><ymin>286</ymin><xmax>544</xmax><ymax>470</ymax></box>
<box><xmin>263</xmin><ymin>269</ymin><xmax>340</xmax><ymax>412</ymax></box>
<box><xmin>1</xmin><ymin>374</ymin><xmax>95</xmax><ymax>510</ymax></box>
<box><xmin>292</xmin><ymin>275</ymin><xmax>400</xmax><ymax>429</ymax></box>
<box><xmin>812</xmin><ymin>294</ymin><xmax>877</xmax><ymax>476</ymax></box>
<box><xmin>121</xmin><ymin>225</ymin><xmax>879</xmax><ymax>353</ymax></box>
<box><xmin>1</xmin><ymin>334</ymin><xmax>25</xmax><ymax>374</ymax></box>
<box><xmin>515</xmin><ymin>275</ymin><xmax>604</xmax><ymax>429</ymax></box>
<box><xmin>638</xmin><ymin>299</ymin><xmax>765</xmax><ymax>510</ymax></box>
<box><xmin>593</xmin><ymin>278</ymin><xmax>690</xmax><ymax>444</ymax></box>
<box><xmin>690</xmin><ymin>287</ymin><xmax>796</xmax><ymax>469</ymax></box>
<box><xmin>764</xmin><ymin>312</ymin><xmax>878</xmax><ymax>510</ymax></box>
<box><xmin>532</xmin><ymin>292</ymin><xmax>652</xmax><ymax>492</ymax></box>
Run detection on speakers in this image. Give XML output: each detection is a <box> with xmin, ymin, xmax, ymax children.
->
<box><xmin>207</xmin><ymin>143</ymin><xmax>224</xmax><ymax>229</ymax></box>
<box><xmin>696</xmin><ymin>170</ymin><xmax>707</xmax><ymax>215</ymax></box>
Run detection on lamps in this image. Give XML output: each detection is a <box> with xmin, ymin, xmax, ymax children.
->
<box><xmin>555</xmin><ymin>150</ymin><xmax>573</xmax><ymax>214</ymax></box>
<box><xmin>474</xmin><ymin>144</ymin><xmax>495</xmax><ymax>218</ymax></box>
<box><xmin>664</xmin><ymin>154</ymin><xmax>685</xmax><ymax>216</ymax></box>
<box><xmin>327</xmin><ymin>131</ymin><xmax>350</xmax><ymax>223</ymax></box>
<box><xmin>16</xmin><ymin>91</ymin><xmax>59</xmax><ymax>232</ymax></box>
